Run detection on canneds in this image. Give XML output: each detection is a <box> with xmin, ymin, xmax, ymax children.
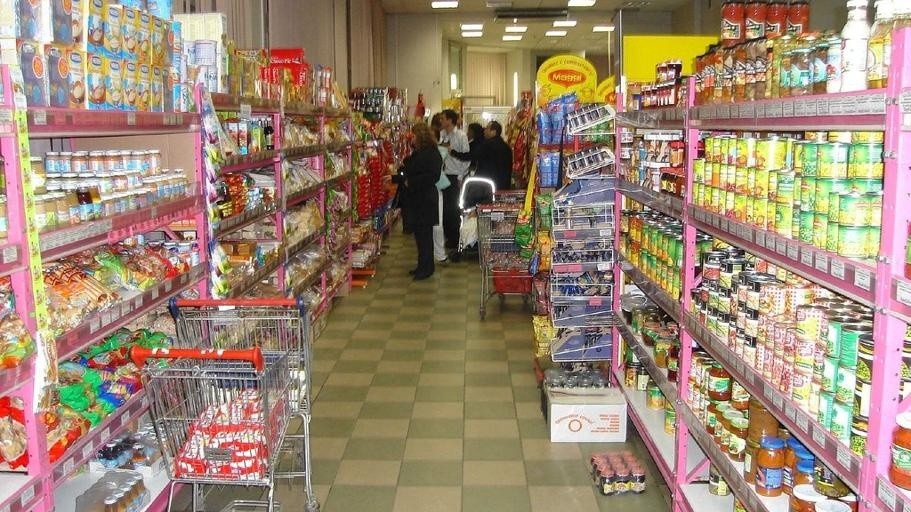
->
<box><xmin>543</xmin><ymin>0</ymin><xmax>910</xmax><ymax>512</ymax></box>
<box><xmin>0</xmin><ymin>150</ymin><xmax>191</xmax><ymax>240</ymax></box>
<box><xmin>0</xmin><ymin>149</ymin><xmax>190</xmax><ymax>241</ymax></box>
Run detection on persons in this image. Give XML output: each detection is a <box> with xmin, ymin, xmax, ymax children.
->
<box><xmin>387</xmin><ymin>111</ymin><xmax>513</xmax><ymax>279</ymax></box>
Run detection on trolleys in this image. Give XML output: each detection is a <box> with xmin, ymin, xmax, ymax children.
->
<box><xmin>165</xmin><ymin>294</ymin><xmax>324</xmax><ymax>512</ymax></box>
<box><xmin>129</xmin><ymin>342</ymin><xmax>295</xmax><ymax>512</ymax></box>
<box><xmin>474</xmin><ymin>204</ymin><xmax>536</xmax><ymax>322</ymax></box>
<box><xmin>490</xmin><ymin>187</ymin><xmax>533</xmax><ymax>204</ymax></box>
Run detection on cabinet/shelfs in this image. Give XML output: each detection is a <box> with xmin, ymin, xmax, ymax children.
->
<box><xmin>524</xmin><ymin>26</ymin><xmax>911</xmax><ymax>512</ymax></box>
<box><xmin>0</xmin><ymin>63</ymin><xmax>412</xmax><ymax>512</ymax></box>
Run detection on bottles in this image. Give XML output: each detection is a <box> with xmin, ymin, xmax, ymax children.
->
<box><xmin>543</xmin><ymin>370</ymin><xmax>612</xmax><ymax>424</ymax></box>
<box><xmin>75</xmin><ymin>417</ymin><xmax>188</xmax><ymax>511</ymax></box>
<box><xmin>311</xmin><ymin>63</ymin><xmax>332</xmax><ymax>110</ymax></box>
<box><xmin>350</xmin><ymin>87</ymin><xmax>408</xmax><ymax>126</ymax></box>
<box><xmin>840</xmin><ymin>1</ymin><xmax>911</xmax><ymax>91</ymax></box>
<box><xmin>0</xmin><ymin>157</ymin><xmax>103</xmax><ymax>242</ymax></box>
<box><xmin>251</xmin><ymin>116</ymin><xmax>275</xmax><ymax>154</ymax></box>
